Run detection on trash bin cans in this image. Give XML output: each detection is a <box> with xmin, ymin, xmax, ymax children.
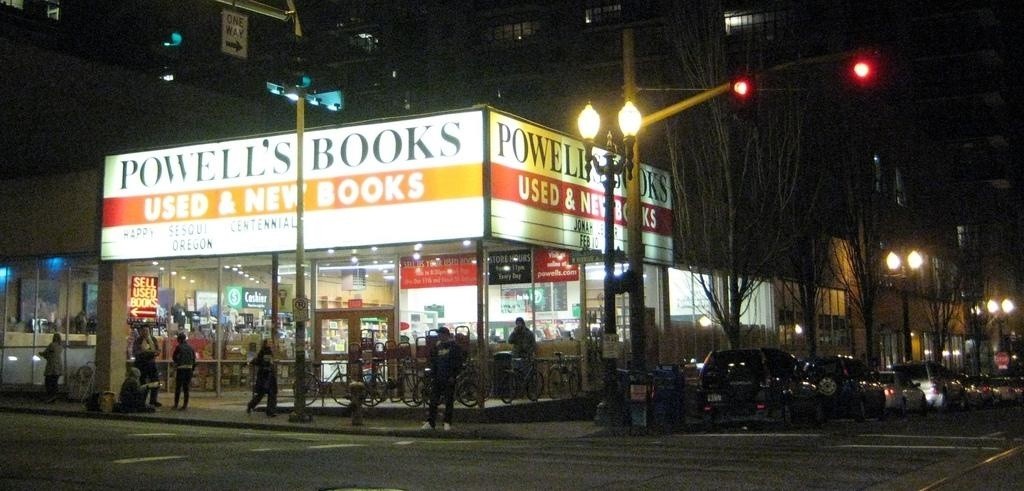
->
<box><xmin>494</xmin><ymin>351</ymin><xmax>513</xmax><ymax>394</ymax></box>
<box><xmin>615</xmin><ymin>367</ymin><xmax>655</xmax><ymax>436</ymax></box>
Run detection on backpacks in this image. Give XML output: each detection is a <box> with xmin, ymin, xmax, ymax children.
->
<box><xmin>85</xmin><ymin>390</ymin><xmax>100</xmax><ymax>411</ymax></box>
<box><xmin>99</xmin><ymin>391</ymin><xmax>116</xmax><ymax>412</ymax></box>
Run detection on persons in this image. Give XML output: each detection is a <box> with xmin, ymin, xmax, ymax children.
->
<box><xmin>38</xmin><ymin>333</ymin><xmax>63</xmax><ymax>403</ymax></box>
<box><xmin>43</xmin><ymin>309</ymin><xmax>96</xmax><ymax>336</ymax></box>
<box><xmin>419</xmin><ymin>327</ymin><xmax>462</xmax><ymax>432</ymax></box>
<box><xmin>245</xmin><ymin>338</ymin><xmax>278</xmax><ymax>419</ymax></box>
<box><xmin>171</xmin><ymin>332</ymin><xmax>196</xmax><ymax>409</ymax></box>
<box><xmin>508</xmin><ymin>317</ymin><xmax>536</xmax><ymax>365</ymax></box>
<box><xmin>849</xmin><ymin>352</ymin><xmax>879</xmax><ymax>366</ymax></box>
<box><xmin>554</xmin><ymin>320</ymin><xmax>565</xmax><ymax>336</ymax></box>
<box><xmin>119</xmin><ymin>367</ymin><xmax>162</xmax><ymax>413</ymax></box>
<box><xmin>489</xmin><ymin>329</ymin><xmax>500</xmax><ymax>342</ymax></box>
<box><xmin>132</xmin><ymin>324</ymin><xmax>162</xmax><ymax>407</ymax></box>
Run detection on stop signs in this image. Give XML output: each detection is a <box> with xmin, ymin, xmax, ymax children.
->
<box><xmin>995</xmin><ymin>352</ymin><xmax>1010</xmax><ymax>366</ymax></box>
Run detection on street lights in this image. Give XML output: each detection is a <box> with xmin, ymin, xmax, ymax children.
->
<box><xmin>886</xmin><ymin>250</ymin><xmax>923</xmax><ymax>359</ymax></box>
<box><xmin>260</xmin><ymin>69</ymin><xmax>342</xmax><ymax>425</ymax></box>
<box><xmin>986</xmin><ymin>298</ymin><xmax>1014</xmax><ymax>371</ymax></box>
<box><xmin>576</xmin><ymin>99</ymin><xmax>642</xmax><ymax>435</ymax></box>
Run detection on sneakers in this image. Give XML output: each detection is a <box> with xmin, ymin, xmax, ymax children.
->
<box><xmin>421</xmin><ymin>421</ymin><xmax>435</xmax><ymax>430</ymax></box>
<box><xmin>148</xmin><ymin>401</ymin><xmax>187</xmax><ymax>410</ymax></box>
<box><xmin>246</xmin><ymin>403</ymin><xmax>255</xmax><ymax>414</ymax></box>
<box><xmin>267</xmin><ymin>411</ymin><xmax>279</xmax><ymax>418</ymax></box>
<box><xmin>443</xmin><ymin>422</ymin><xmax>452</xmax><ymax>431</ymax></box>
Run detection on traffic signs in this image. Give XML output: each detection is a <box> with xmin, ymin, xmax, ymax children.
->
<box><xmin>219</xmin><ymin>8</ymin><xmax>249</xmax><ymax>60</ymax></box>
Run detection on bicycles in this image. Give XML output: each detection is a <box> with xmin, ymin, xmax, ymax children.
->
<box><xmin>291</xmin><ymin>350</ymin><xmax>587</xmax><ymax>408</ymax></box>
